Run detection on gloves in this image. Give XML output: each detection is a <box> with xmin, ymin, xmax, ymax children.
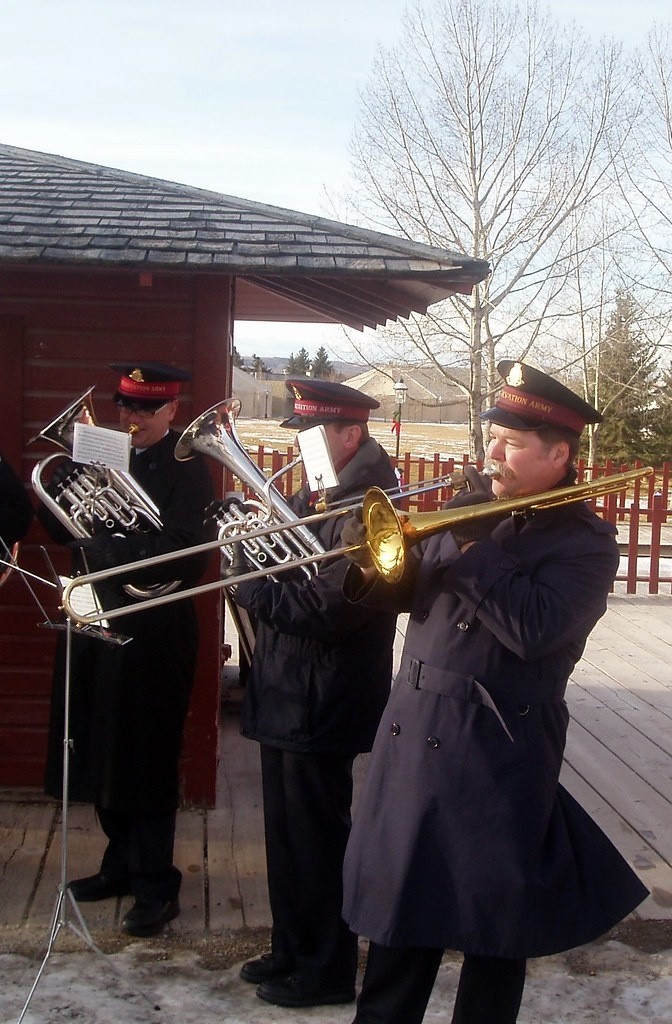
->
<box><xmin>340</xmin><ymin>506</ymin><xmax>375</xmax><ymax>569</ymax></box>
<box><xmin>442</xmin><ymin>465</ymin><xmax>511</xmax><ymax>550</ymax></box>
<box><xmin>45</xmin><ymin>460</ymin><xmax>84</xmax><ymax>498</ymax></box>
<box><xmin>67</xmin><ymin>516</ymin><xmax>130</xmax><ymax>566</ymax></box>
<box><xmin>203</xmin><ymin>497</ymin><xmax>252</xmax><ymax>524</ymax></box>
<box><xmin>219</xmin><ymin>541</ymin><xmax>253</xmax><ymax>602</ymax></box>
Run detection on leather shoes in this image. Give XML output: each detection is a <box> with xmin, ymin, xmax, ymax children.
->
<box><xmin>67</xmin><ymin>873</ymin><xmax>136</xmax><ymax>901</ymax></box>
<box><xmin>256</xmin><ymin>975</ymin><xmax>356</xmax><ymax>1007</ymax></box>
<box><xmin>120</xmin><ymin>900</ymin><xmax>180</xmax><ymax>937</ymax></box>
<box><xmin>240</xmin><ymin>954</ymin><xmax>292</xmax><ymax>983</ymax></box>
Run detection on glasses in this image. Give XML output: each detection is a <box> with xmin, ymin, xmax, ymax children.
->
<box><xmin>117</xmin><ymin>401</ymin><xmax>173</xmax><ymax>419</ymax></box>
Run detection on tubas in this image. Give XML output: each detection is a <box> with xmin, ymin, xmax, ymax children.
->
<box><xmin>31</xmin><ymin>383</ymin><xmax>182</xmax><ymax>599</ymax></box>
<box><xmin>174</xmin><ymin>396</ymin><xmax>328</xmax><ymax>586</ymax></box>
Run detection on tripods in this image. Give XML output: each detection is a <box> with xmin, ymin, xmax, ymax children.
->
<box><xmin>0</xmin><ymin>537</ymin><xmax>160</xmax><ymax>1024</ymax></box>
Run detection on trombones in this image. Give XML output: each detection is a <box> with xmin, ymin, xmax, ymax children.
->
<box><xmin>54</xmin><ymin>460</ymin><xmax>658</xmax><ymax>624</ymax></box>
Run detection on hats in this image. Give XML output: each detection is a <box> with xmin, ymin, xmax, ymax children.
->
<box><xmin>479</xmin><ymin>360</ymin><xmax>604</xmax><ymax>439</ymax></box>
<box><xmin>280</xmin><ymin>379</ymin><xmax>380</xmax><ymax>429</ymax></box>
<box><xmin>110</xmin><ymin>360</ymin><xmax>192</xmax><ymax>410</ymax></box>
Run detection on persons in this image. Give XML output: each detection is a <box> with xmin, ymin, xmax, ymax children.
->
<box><xmin>220</xmin><ymin>379</ymin><xmax>402</xmax><ymax>1008</ymax></box>
<box><xmin>37</xmin><ymin>361</ymin><xmax>214</xmax><ymax>936</ymax></box>
<box><xmin>342</xmin><ymin>359</ymin><xmax>651</xmax><ymax>1024</ymax></box>
<box><xmin>0</xmin><ymin>453</ymin><xmax>35</xmax><ymax>554</ymax></box>
